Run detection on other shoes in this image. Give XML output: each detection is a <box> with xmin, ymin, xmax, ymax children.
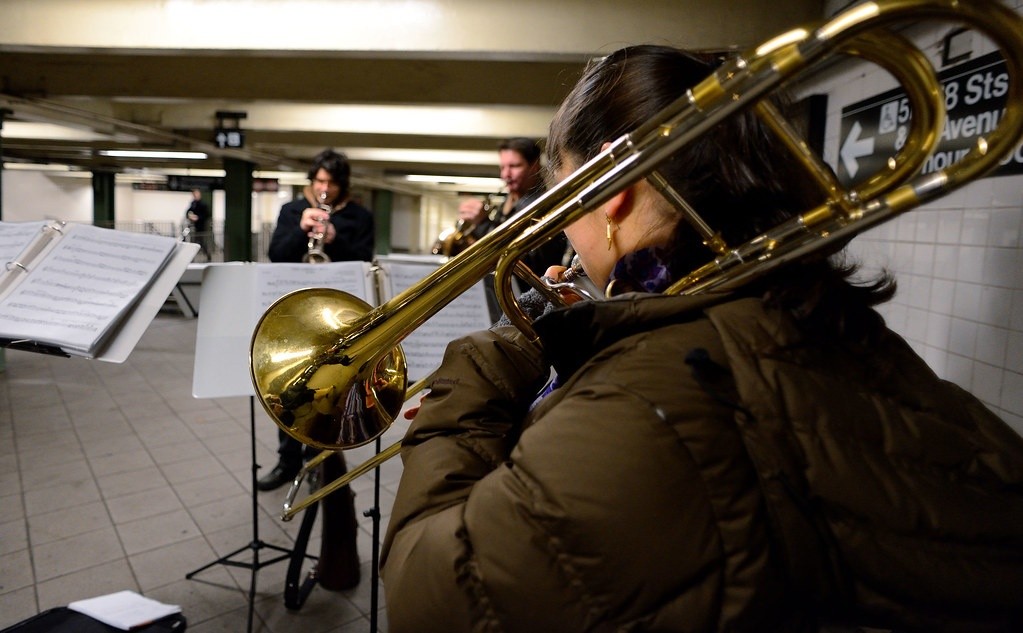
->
<box><xmin>258</xmin><ymin>467</ymin><xmax>291</xmax><ymax>490</ymax></box>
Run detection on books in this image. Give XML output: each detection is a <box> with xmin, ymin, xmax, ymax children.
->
<box><xmin>253</xmin><ymin>257</ymin><xmax>492</xmax><ymax>381</ymax></box>
<box><xmin>0</xmin><ymin>222</ymin><xmax>179</xmax><ymax>360</ymax></box>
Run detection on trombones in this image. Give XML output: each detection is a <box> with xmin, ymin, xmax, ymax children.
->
<box><xmin>248</xmin><ymin>0</ymin><xmax>1023</xmax><ymax>521</ymax></box>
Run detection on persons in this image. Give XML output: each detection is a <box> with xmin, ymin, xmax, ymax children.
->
<box><xmin>452</xmin><ymin>137</ymin><xmax>566</xmax><ymax>324</ymax></box>
<box><xmin>258</xmin><ymin>150</ymin><xmax>376</xmax><ymax>491</ymax></box>
<box><xmin>380</xmin><ymin>46</ymin><xmax>921</xmax><ymax>633</ymax></box>
<box><xmin>185</xmin><ymin>189</ymin><xmax>211</xmax><ymax>263</ymax></box>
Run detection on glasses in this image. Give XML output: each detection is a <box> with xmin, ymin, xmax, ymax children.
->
<box><xmin>315</xmin><ymin>177</ymin><xmax>337</xmax><ymax>186</ymax></box>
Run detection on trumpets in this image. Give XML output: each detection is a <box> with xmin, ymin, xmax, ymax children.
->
<box><xmin>301</xmin><ymin>191</ymin><xmax>332</xmax><ymax>263</ymax></box>
<box><xmin>433</xmin><ymin>178</ymin><xmax>512</xmax><ymax>259</ymax></box>
